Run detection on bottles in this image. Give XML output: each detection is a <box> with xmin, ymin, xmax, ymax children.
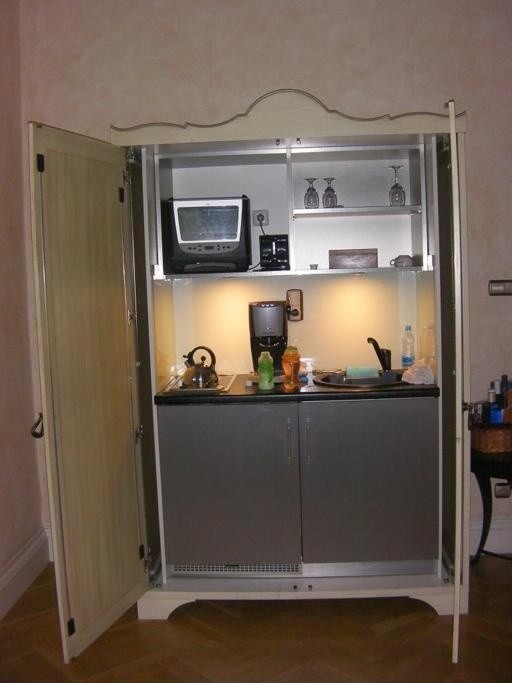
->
<box><xmin>402</xmin><ymin>326</ymin><xmax>415</xmax><ymax>370</ymax></box>
<box><xmin>282</xmin><ymin>345</ymin><xmax>301</xmax><ymax>389</ymax></box>
<box><xmin>258</xmin><ymin>351</ymin><xmax>275</xmax><ymax>390</ymax></box>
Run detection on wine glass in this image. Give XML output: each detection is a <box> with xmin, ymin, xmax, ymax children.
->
<box><xmin>322</xmin><ymin>177</ymin><xmax>337</xmax><ymax>207</ymax></box>
<box><xmin>387</xmin><ymin>165</ymin><xmax>405</xmax><ymax>206</ymax></box>
<box><xmin>304</xmin><ymin>178</ymin><xmax>319</xmax><ymax>208</ymax></box>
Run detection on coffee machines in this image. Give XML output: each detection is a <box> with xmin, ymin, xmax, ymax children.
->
<box><xmin>248</xmin><ymin>301</ymin><xmax>294</xmax><ymax>383</ymax></box>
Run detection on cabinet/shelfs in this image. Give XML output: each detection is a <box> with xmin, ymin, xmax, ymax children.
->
<box><xmin>140</xmin><ymin>134</ymin><xmax>451</xmax><ymax>282</ymax></box>
<box><xmin>157</xmin><ymin>396</ymin><xmax>440</xmax><ymax>573</ymax></box>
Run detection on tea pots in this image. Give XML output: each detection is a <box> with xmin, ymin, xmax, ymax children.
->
<box><xmin>181</xmin><ymin>346</ymin><xmax>219</xmax><ymax>388</ymax></box>
<box><xmin>389</xmin><ymin>254</ymin><xmax>417</xmax><ymax>267</ymax></box>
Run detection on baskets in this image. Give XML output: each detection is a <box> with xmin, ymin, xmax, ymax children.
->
<box><xmin>471</xmin><ymin>423</ymin><xmax>512</xmax><ymax>453</ymax></box>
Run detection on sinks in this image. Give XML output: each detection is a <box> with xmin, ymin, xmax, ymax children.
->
<box><xmin>313</xmin><ymin>372</ymin><xmax>411</xmax><ymax>388</ymax></box>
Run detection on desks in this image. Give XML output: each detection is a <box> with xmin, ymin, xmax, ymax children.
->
<box><xmin>468</xmin><ymin>448</ymin><xmax>512</xmax><ymax>565</ymax></box>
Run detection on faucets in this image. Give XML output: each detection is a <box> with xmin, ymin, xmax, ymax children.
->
<box><xmin>366</xmin><ymin>337</ymin><xmax>392</xmax><ymax>372</ymax></box>
